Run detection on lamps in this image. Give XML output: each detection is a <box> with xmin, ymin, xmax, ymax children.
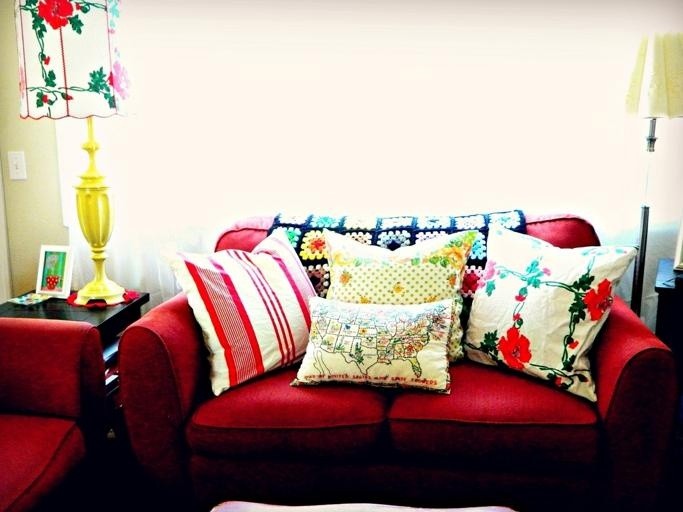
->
<box><xmin>12</xmin><ymin>1</ymin><xmax>141</xmax><ymax>311</ymax></box>
<box><xmin>626</xmin><ymin>29</ymin><xmax>682</xmax><ymax>319</ymax></box>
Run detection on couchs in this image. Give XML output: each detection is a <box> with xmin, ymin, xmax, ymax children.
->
<box><xmin>117</xmin><ymin>216</ymin><xmax>681</xmax><ymax>512</ymax></box>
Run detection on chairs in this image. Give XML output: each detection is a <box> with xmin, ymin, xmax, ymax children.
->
<box><xmin>1</xmin><ymin>318</ymin><xmax>109</xmax><ymax>510</ymax></box>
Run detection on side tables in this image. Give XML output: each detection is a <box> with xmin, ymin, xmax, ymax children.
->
<box><xmin>0</xmin><ymin>289</ymin><xmax>149</xmax><ymax>464</ymax></box>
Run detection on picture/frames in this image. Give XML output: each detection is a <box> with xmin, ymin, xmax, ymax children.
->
<box><xmin>35</xmin><ymin>243</ymin><xmax>76</xmax><ymax>300</ymax></box>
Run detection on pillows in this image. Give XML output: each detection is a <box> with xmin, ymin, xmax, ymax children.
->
<box><xmin>461</xmin><ymin>223</ymin><xmax>641</xmax><ymax>403</ymax></box>
<box><xmin>289</xmin><ymin>297</ymin><xmax>456</xmax><ymax>397</ymax></box>
<box><xmin>319</xmin><ymin>228</ymin><xmax>479</xmax><ymax>366</ymax></box>
<box><xmin>163</xmin><ymin>226</ymin><xmax>326</xmax><ymax>396</ymax></box>
